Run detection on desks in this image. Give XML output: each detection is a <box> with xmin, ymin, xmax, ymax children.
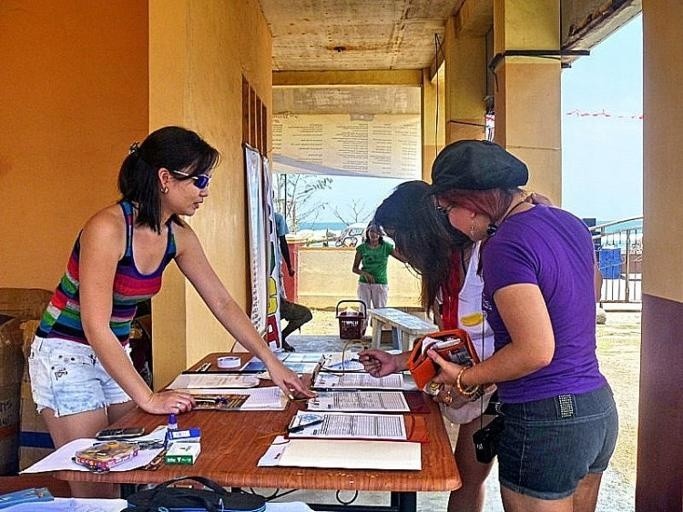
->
<box><xmin>50</xmin><ymin>349</ymin><xmax>463</xmax><ymax>512</ymax></box>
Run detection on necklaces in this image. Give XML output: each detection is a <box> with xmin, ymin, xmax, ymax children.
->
<box><xmin>499</xmin><ymin>199</ymin><xmax>525</xmax><ymax>223</ymax></box>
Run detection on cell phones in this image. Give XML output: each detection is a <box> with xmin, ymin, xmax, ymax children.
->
<box><xmin>95</xmin><ymin>426</ymin><xmax>145</xmax><ymax>438</ymax></box>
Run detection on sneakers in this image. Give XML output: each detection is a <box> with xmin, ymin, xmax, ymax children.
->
<box><xmin>276</xmin><ymin>340</ymin><xmax>295</xmax><ymax>352</ymax></box>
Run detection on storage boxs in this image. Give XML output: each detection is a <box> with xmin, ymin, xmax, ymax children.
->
<box><xmin>15</xmin><ymin>319</ymin><xmax>56</xmax><ymax>472</ymax></box>
<box><xmin>1</xmin><ymin>315</ymin><xmax>28</xmax><ymax>474</ymax></box>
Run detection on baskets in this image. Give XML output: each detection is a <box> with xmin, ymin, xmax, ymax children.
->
<box><xmin>334</xmin><ymin>299</ymin><xmax>369</xmax><ymax>339</ymax></box>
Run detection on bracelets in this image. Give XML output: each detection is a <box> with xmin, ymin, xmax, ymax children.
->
<box><xmin>454</xmin><ymin>366</ymin><xmax>480</xmax><ymax>398</ymax></box>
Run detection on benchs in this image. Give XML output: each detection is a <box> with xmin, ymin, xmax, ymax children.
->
<box><xmin>366</xmin><ymin>307</ymin><xmax>438</xmax><ymax>354</ymax></box>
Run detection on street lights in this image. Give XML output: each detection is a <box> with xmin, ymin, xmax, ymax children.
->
<box><xmin>279</xmin><ymin>173</ymin><xmax>287</xmax><ymax>224</ymax></box>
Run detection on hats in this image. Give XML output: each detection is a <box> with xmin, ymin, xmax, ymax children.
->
<box><xmin>430</xmin><ymin>139</ymin><xmax>527</xmax><ymax>194</ymax></box>
<box><xmin>438</xmin><ymin>384</ymin><xmax>496</xmax><ymax>425</ymax></box>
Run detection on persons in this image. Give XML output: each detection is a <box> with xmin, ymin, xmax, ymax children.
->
<box><xmin>274</xmin><ymin>190</ymin><xmax>295</xmax><ymax>279</ymax></box>
<box><xmin>280</xmin><ymin>295</ymin><xmax>313</xmax><ymax>352</ymax></box>
<box><xmin>422</xmin><ymin>139</ymin><xmax>619</xmax><ymax>512</ymax></box>
<box><xmin>30</xmin><ymin>124</ymin><xmax>317</xmax><ymax>499</ymax></box>
<box><xmin>352</xmin><ymin>220</ymin><xmax>406</xmax><ymax>326</ymax></box>
<box><xmin>355</xmin><ymin>178</ymin><xmax>502</xmax><ymax>511</ymax></box>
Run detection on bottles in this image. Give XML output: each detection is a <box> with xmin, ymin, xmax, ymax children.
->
<box><xmin>167</xmin><ymin>414</ymin><xmax>178</xmax><ymax>433</ymax></box>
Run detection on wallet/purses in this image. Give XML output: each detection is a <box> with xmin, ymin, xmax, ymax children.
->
<box><xmin>407</xmin><ymin>327</ymin><xmax>481</xmax><ymax>388</ymax></box>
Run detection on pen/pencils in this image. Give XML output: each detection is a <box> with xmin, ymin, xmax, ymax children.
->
<box><xmin>364</xmin><ymin>346</ymin><xmax>372</xmax><ymax>363</ymax></box>
<box><xmin>194</xmin><ymin>362</ymin><xmax>211</xmax><ymax>372</ymax></box>
<box><xmin>288</xmin><ymin>420</ymin><xmax>324</xmax><ymax>433</ymax></box>
<box><xmin>314</xmin><ymin>388</ymin><xmax>357</xmax><ymax>392</ymax></box>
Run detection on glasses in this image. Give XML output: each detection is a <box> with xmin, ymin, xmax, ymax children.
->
<box><xmin>436</xmin><ymin>202</ymin><xmax>454</xmax><ymax>217</ymax></box>
<box><xmin>172</xmin><ymin>169</ymin><xmax>209</xmax><ymax>189</ymax></box>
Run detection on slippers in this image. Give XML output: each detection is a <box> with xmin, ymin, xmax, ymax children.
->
<box><xmin>121</xmin><ymin>493</ymin><xmax>217</xmax><ymax>512</ymax></box>
<box><xmin>126</xmin><ymin>474</ymin><xmax>266</xmax><ymax>512</ymax></box>
